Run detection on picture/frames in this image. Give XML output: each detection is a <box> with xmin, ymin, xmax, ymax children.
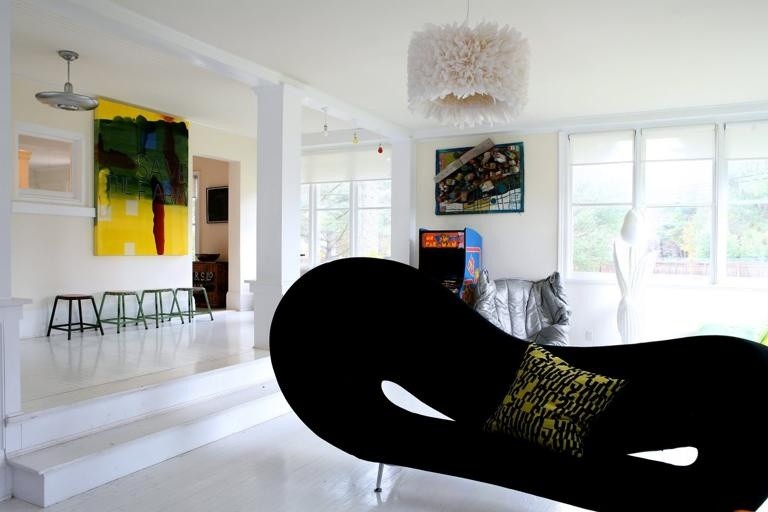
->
<box><xmin>434</xmin><ymin>141</ymin><xmax>527</xmax><ymax>217</ymax></box>
<box><xmin>205</xmin><ymin>186</ymin><xmax>228</xmax><ymax>224</ymax></box>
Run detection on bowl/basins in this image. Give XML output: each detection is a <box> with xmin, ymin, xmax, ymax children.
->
<box><xmin>195</xmin><ymin>253</ymin><xmax>220</xmax><ymax>262</ymax></box>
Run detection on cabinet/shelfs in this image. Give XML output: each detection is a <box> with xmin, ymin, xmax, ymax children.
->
<box><xmin>191</xmin><ymin>260</ymin><xmax>228</xmax><ymax>309</ymax></box>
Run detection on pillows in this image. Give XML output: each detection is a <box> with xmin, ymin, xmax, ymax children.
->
<box><xmin>478</xmin><ymin>339</ymin><xmax>629</xmax><ymax>461</ymax></box>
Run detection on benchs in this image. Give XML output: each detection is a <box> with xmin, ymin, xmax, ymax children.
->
<box><xmin>266</xmin><ymin>254</ymin><xmax>768</xmax><ymax>512</ymax></box>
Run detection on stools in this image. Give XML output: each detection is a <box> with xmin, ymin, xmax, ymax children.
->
<box><xmin>46</xmin><ymin>293</ymin><xmax>104</xmax><ymax>341</ymax></box>
<box><xmin>135</xmin><ymin>289</ymin><xmax>184</xmax><ymax>327</ymax></box>
<box><xmin>94</xmin><ymin>290</ymin><xmax>151</xmax><ymax>334</ymax></box>
<box><xmin>172</xmin><ymin>285</ymin><xmax>216</xmax><ymax>323</ymax></box>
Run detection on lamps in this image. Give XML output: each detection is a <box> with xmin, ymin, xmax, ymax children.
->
<box><xmin>377</xmin><ymin>143</ymin><xmax>384</xmax><ymax>154</ymax></box>
<box><xmin>320</xmin><ymin>104</ymin><xmax>332</xmax><ymax>138</ymax></box>
<box><xmin>403</xmin><ymin>1</ymin><xmax>534</xmax><ymax>131</ymax></box>
<box><xmin>352</xmin><ymin>132</ymin><xmax>359</xmax><ymax>144</ymax></box>
<box><xmin>32</xmin><ymin>48</ymin><xmax>102</xmax><ymax>111</ymax></box>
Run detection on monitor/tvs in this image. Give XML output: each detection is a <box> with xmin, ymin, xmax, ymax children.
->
<box><xmin>425</xmin><ymin>249</ymin><xmax>464</xmax><ymax>281</ymax></box>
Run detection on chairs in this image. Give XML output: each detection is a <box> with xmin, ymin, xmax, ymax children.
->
<box><xmin>469</xmin><ymin>271</ymin><xmax>573</xmax><ymax>347</ymax></box>
